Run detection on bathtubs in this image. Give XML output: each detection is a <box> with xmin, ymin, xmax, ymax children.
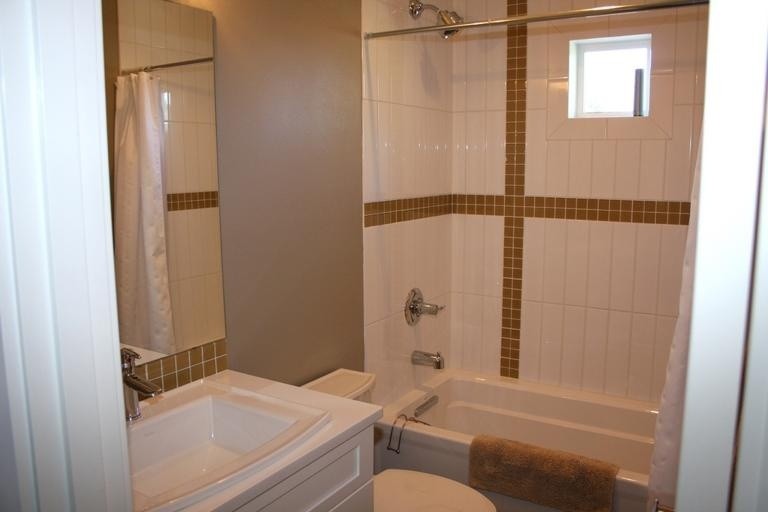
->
<box><xmin>375</xmin><ymin>365</ymin><xmax>660</xmax><ymax>510</ymax></box>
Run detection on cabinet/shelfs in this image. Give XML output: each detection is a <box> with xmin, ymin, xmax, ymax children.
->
<box><xmin>238</xmin><ymin>428</ymin><xmax>375</xmax><ymax>510</ymax></box>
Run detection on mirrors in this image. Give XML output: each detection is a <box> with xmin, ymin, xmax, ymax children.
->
<box><xmin>101</xmin><ymin>0</ymin><xmax>227</xmax><ymax>370</ymax></box>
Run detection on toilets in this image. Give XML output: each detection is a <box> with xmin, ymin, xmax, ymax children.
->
<box><xmin>297</xmin><ymin>368</ymin><xmax>498</xmax><ymax>512</ymax></box>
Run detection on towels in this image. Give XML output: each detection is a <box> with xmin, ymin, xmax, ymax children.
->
<box><xmin>465</xmin><ymin>433</ymin><xmax>619</xmax><ymax>511</ymax></box>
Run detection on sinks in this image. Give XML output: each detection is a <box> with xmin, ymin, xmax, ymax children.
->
<box><xmin>129</xmin><ymin>378</ymin><xmax>331</xmax><ymax>512</ymax></box>
<box><xmin>120</xmin><ymin>344</ymin><xmax>170</xmax><ymax>369</ymax></box>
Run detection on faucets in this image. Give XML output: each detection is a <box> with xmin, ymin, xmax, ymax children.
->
<box><xmin>118</xmin><ymin>346</ymin><xmax>162</xmax><ymax>419</ymax></box>
<box><xmin>411</xmin><ymin>347</ymin><xmax>445</xmax><ymax>373</ymax></box>
